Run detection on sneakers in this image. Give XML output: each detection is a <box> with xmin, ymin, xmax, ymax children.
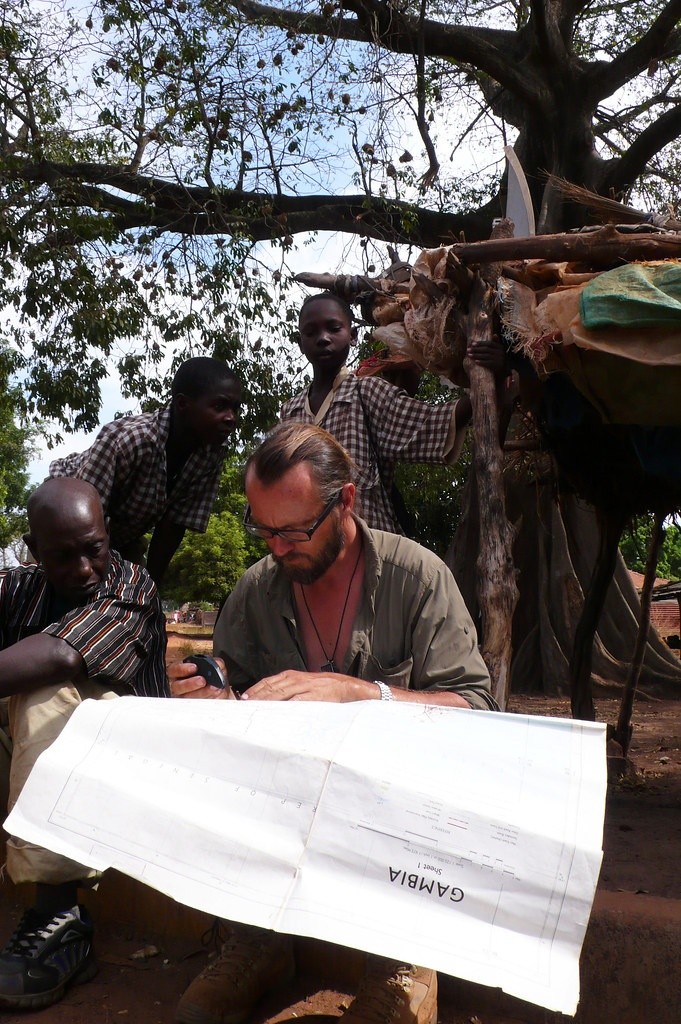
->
<box><xmin>174</xmin><ymin>920</ymin><xmax>302</xmax><ymax>1024</ymax></box>
<box><xmin>338</xmin><ymin>952</ymin><xmax>439</xmax><ymax>1024</ymax></box>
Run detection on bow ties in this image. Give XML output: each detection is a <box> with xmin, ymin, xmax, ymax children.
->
<box><xmin>0</xmin><ymin>905</ymin><xmax>97</xmax><ymax>1007</ymax></box>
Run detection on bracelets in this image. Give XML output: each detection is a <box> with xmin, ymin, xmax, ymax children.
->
<box><xmin>374</xmin><ymin>680</ymin><xmax>393</xmax><ymax>702</ymax></box>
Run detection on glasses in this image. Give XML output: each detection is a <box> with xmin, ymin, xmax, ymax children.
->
<box><xmin>243</xmin><ymin>489</ymin><xmax>343</xmax><ymax>542</ymax></box>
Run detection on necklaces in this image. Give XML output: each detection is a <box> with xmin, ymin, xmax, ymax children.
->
<box><xmin>299</xmin><ymin>532</ymin><xmax>363</xmax><ymax>672</ymax></box>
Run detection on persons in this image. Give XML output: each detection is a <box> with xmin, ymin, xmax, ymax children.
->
<box><xmin>47</xmin><ymin>356</ymin><xmax>244</xmax><ymax>590</ymax></box>
<box><xmin>0</xmin><ymin>476</ymin><xmax>175</xmax><ymax>1007</ymax></box>
<box><xmin>167</xmin><ymin>422</ymin><xmax>500</xmax><ymax>1023</ymax></box>
<box><xmin>283</xmin><ymin>295</ymin><xmax>512</xmax><ymax>534</ymax></box>
